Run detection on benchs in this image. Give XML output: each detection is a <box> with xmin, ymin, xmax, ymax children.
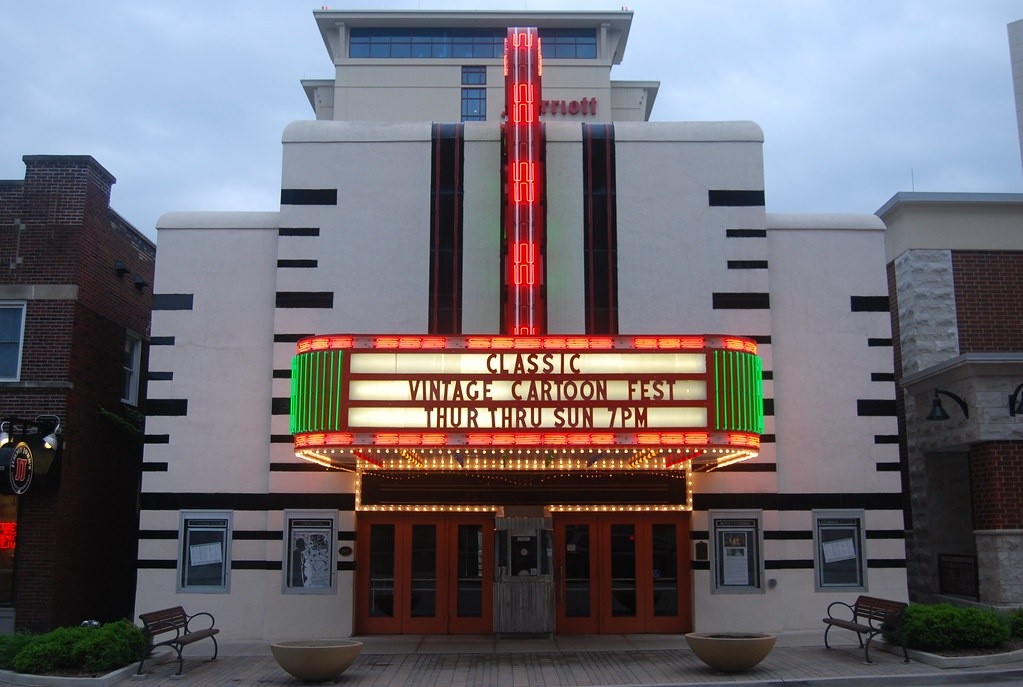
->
<box><xmin>137</xmin><ymin>606</ymin><xmax>219</xmax><ymax>676</ymax></box>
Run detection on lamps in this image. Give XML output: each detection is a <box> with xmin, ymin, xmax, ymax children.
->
<box><xmin>35</xmin><ymin>414</ymin><xmax>60</xmax><ymax>451</ymax></box>
<box><xmin>0</xmin><ymin>421</ymin><xmax>38</xmax><ymax>449</ymax></box>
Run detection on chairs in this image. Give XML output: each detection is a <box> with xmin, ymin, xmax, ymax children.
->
<box><xmin>823</xmin><ymin>595</ymin><xmax>910</xmax><ymax>664</ymax></box>
